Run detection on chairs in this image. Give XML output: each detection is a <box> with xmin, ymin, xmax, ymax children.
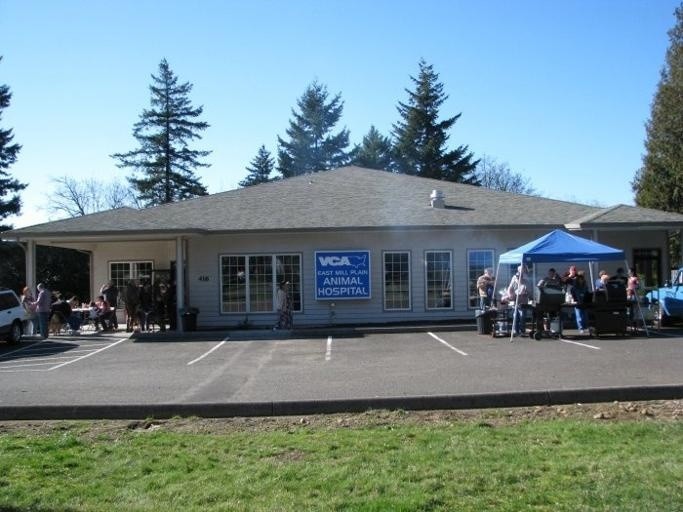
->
<box><xmin>516</xmin><ymin>303</ymin><xmax>537</xmax><ymax>336</ymax></box>
<box><xmin>48</xmin><ymin>299</ymin><xmax>116</xmax><ymax>336</ymax></box>
<box><xmin>490</xmin><ymin>308</ymin><xmax>520</xmax><ymax>337</ymax></box>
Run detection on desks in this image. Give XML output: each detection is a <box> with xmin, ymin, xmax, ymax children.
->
<box><xmin>532</xmin><ymin>300</ymin><xmax>636</xmax><ymax>340</ymax></box>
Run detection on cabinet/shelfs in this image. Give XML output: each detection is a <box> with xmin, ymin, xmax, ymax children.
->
<box><xmin>630</xmin><ymin>287</ymin><xmax>661</xmax><ymax>337</ymax></box>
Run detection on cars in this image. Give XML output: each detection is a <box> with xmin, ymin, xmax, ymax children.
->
<box><xmin>645</xmin><ymin>267</ymin><xmax>682</xmax><ymax>327</ymax></box>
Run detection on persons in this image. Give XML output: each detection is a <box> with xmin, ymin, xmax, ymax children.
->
<box><xmin>20</xmin><ymin>273</ymin><xmax>185</xmax><ymax>342</ymax></box>
<box><xmin>273</xmin><ymin>281</ymin><xmax>294</xmax><ymax>330</ymax></box>
<box><xmin>506</xmin><ymin>266</ymin><xmax>640</xmax><ymax>337</ymax></box>
<box><xmin>477</xmin><ymin>268</ymin><xmax>497</xmax><ymax>309</ymax></box>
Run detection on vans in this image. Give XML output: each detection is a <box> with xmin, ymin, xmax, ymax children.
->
<box><xmin>1</xmin><ymin>287</ymin><xmax>29</xmax><ymax>345</ymax></box>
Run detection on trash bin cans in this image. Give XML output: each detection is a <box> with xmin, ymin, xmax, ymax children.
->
<box><xmin>179</xmin><ymin>308</ymin><xmax>199</xmax><ymax>332</ymax></box>
<box><xmin>475</xmin><ymin>310</ymin><xmax>493</xmax><ymax>335</ymax></box>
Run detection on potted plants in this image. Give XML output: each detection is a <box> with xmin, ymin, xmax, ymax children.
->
<box><xmin>179</xmin><ymin>304</ymin><xmax>199</xmax><ymax>332</ymax></box>
